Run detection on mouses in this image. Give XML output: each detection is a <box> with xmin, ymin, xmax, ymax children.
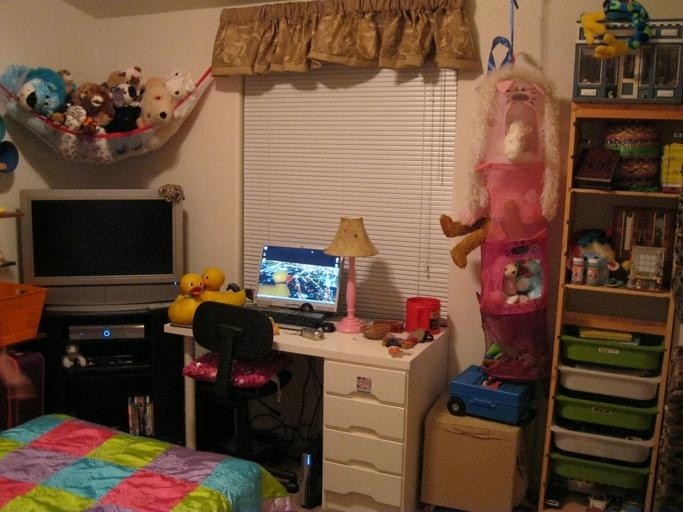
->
<box><xmin>323</xmin><ymin>322</ymin><xmax>335</xmax><ymax>333</ymax></box>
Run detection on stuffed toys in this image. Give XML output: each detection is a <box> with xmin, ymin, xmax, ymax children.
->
<box><xmin>52</xmin><ymin>59</ymin><xmax>145</xmax><ymax>162</ymax></box>
<box><xmin>1</xmin><ymin>64</ymin><xmax>66</xmax><ymax>135</ymax></box>
<box><xmin>502</xmin><ymin>120</ymin><xmax>532</xmax><ymax>161</ymax></box>
<box><xmin>487</xmin><ymin>242</ymin><xmax>548</xmax><ymax>309</ymax></box>
<box><xmin>137</xmin><ymin>77</ymin><xmax>175</xmax><ymax>149</ymax></box>
<box><xmin>170</xmin><ymin>73</ymin><xmax>195</xmax><ymax>98</ymax></box>
<box><xmin>582</xmin><ymin>0</ymin><xmax>649</xmax><ymax>57</ymax></box>
<box><xmin>467</xmin><ymin>66</ymin><xmax>560</xmax><ymax>221</ymax></box>
<box><xmin>441</xmin><ymin>186</ymin><xmax>540</xmax><ymax>267</ymax></box>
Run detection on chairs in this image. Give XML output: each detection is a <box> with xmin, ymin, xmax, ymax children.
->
<box><xmin>192</xmin><ymin>301</ymin><xmax>299</xmax><ymax>493</ymax></box>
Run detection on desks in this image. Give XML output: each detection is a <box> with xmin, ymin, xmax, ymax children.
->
<box><xmin>1</xmin><ymin>277</ymin><xmax>48</xmax><ymax>350</ymax></box>
<box><xmin>164</xmin><ymin>318</ymin><xmax>448</xmax><ymax>512</ymax></box>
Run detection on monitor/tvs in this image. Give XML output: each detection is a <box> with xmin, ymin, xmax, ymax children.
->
<box><xmin>20</xmin><ymin>188</ymin><xmax>184</xmax><ymax>307</ymax></box>
<box><xmin>253</xmin><ymin>242</ymin><xmax>345</xmax><ymax>312</ymax></box>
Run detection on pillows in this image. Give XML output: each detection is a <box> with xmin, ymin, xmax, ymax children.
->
<box><xmin>182</xmin><ymin>353</ymin><xmax>270</xmax><ymax>388</ymax></box>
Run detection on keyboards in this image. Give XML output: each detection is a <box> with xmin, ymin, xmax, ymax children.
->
<box><xmin>242</xmin><ymin>301</ymin><xmax>324</xmax><ymax>332</ymax></box>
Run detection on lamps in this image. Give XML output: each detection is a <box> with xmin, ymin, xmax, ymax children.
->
<box><xmin>324</xmin><ymin>216</ymin><xmax>379</xmax><ymax>334</ymax></box>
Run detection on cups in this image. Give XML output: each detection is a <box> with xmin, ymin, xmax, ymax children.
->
<box><xmin>406</xmin><ymin>297</ymin><xmax>440</xmax><ymax>335</ymax></box>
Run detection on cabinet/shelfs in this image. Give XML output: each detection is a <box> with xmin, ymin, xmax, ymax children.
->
<box><xmin>40</xmin><ymin>303</ymin><xmax>183</xmax><ymax>446</ymax></box>
<box><xmin>538</xmin><ymin>102</ymin><xmax>683</xmax><ymax>511</ymax></box>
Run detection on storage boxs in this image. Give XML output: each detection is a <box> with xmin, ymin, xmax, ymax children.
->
<box><xmin>420</xmin><ymin>393</ymin><xmax>536</xmax><ymax>512</ymax></box>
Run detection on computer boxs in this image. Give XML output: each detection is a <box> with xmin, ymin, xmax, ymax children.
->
<box><xmin>302</xmin><ymin>433</ymin><xmax>322</xmax><ymax>508</ymax></box>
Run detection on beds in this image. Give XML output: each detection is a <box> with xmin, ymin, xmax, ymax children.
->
<box><xmin>0</xmin><ymin>415</ymin><xmax>298</xmax><ymax>512</ymax></box>
